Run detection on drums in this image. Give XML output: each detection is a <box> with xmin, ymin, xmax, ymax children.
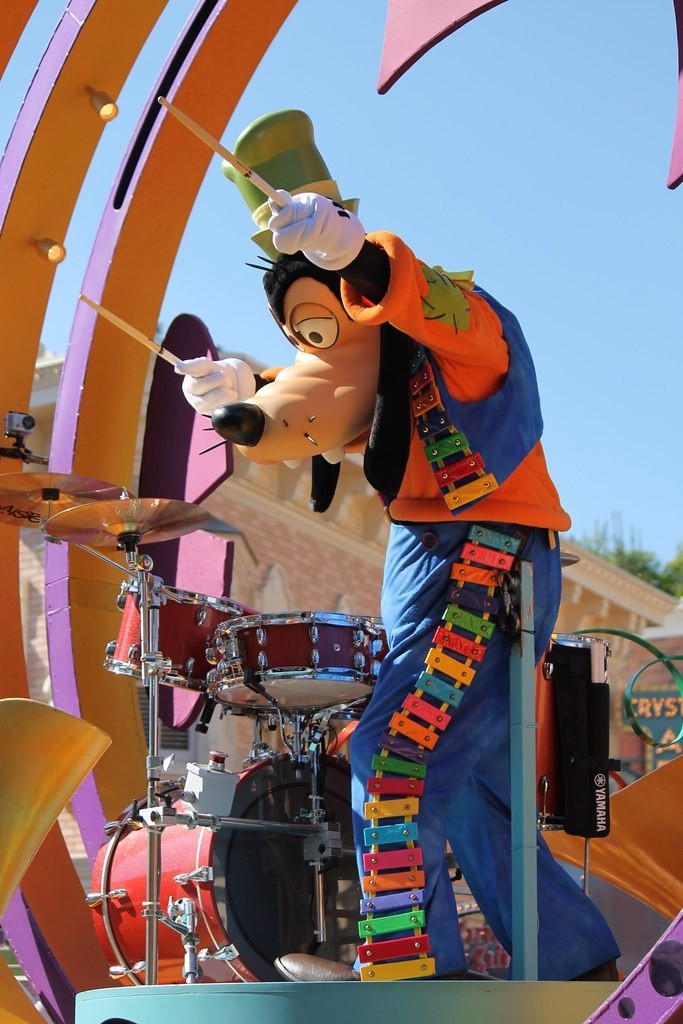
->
<box><xmin>203</xmin><ymin>611</ymin><xmax>376</xmax><ymax>710</ymax></box>
<box><xmin>102</xmin><ymin>575</ymin><xmax>261</xmax><ymax>693</ymax></box>
<box><xmin>86</xmin><ymin>746</ymin><xmax>368</xmax><ymax>985</ymax></box>
<box><xmin>551</xmin><ymin>631</ymin><xmax>612</xmax><ymax>685</ymax></box>
<box><xmin>287</xmin><ymin>615</ymin><xmax>390</xmax><ymax>721</ymax></box>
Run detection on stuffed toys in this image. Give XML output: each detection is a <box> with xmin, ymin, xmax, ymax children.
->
<box><xmin>175</xmin><ymin>190</ymin><xmax>622</xmax><ymax>980</ymax></box>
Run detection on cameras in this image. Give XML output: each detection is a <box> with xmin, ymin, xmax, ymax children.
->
<box><xmin>5</xmin><ymin>412</ymin><xmax>35</xmax><ymax>438</ymax></box>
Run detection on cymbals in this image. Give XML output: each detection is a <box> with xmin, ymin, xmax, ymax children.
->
<box><xmin>560</xmin><ymin>551</ymin><xmax>582</xmax><ymax>569</ymax></box>
<box><xmin>44</xmin><ymin>497</ymin><xmax>210</xmax><ymax>548</ymax></box>
<box><xmin>0</xmin><ymin>471</ymin><xmax>137</xmax><ymax>530</ymax></box>
